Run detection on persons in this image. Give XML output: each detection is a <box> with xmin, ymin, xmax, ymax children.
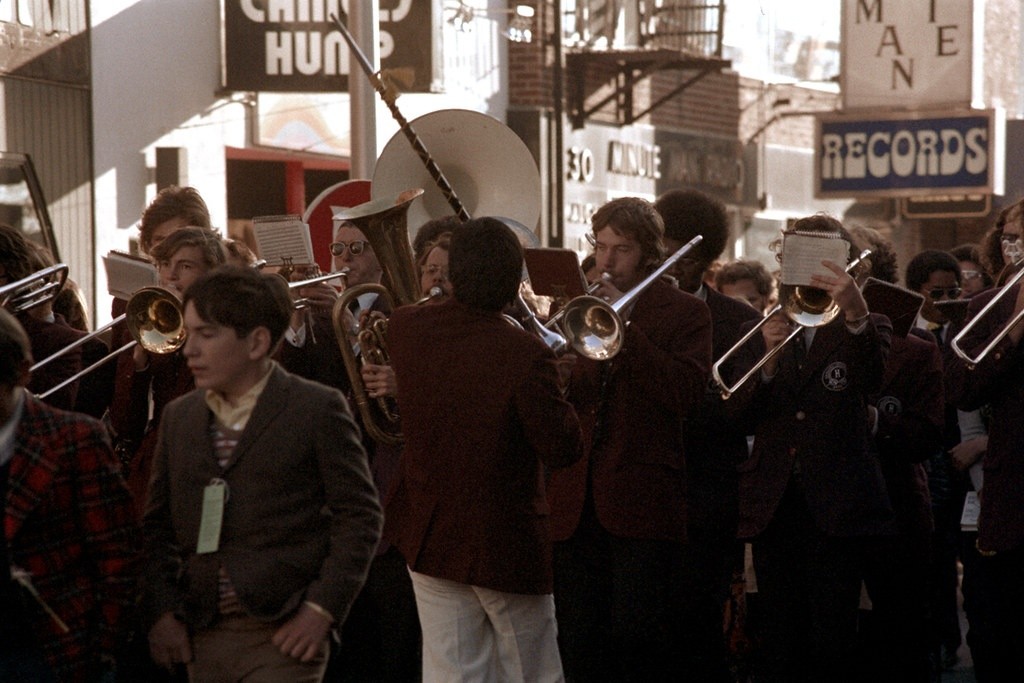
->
<box><xmin>384</xmin><ymin>217</ymin><xmax>588</xmax><ymax>683</ymax></box>
<box><xmin>1</xmin><ymin>184</ymin><xmax>462</xmax><ymax>682</ymax></box>
<box><xmin>555</xmin><ymin>187</ymin><xmax>1024</xmax><ymax>683</ymax></box>
<box><xmin>137</xmin><ymin>262</ymin><xmax>385</xmax><ymax>683</ymax></box>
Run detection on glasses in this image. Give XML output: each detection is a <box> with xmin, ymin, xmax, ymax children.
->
<box><xmin>329</xmin><ymin>239</ymin><xmax>370</xmax><ymax>257</ymax></box>
<box><xmin>959</xmin><ymin>270</ymin><xmax>988</xmax><ymax>281</ymax></box>
<box><xmin>663</xmin><ymin>252</ymin><xmax>701</xmax><ymax>274</ymax></box>
<box><xmin>419</xmin><ymin>263</ymin><xmax>451</xmax><ymax>278</ymax></box>
<box><xmin>1001</xmin><ymin>233</ymin><xmax>1019</xmax><ymax>243</ymax></box>
<box><xmin>923</xmin><ymin>287</ymin><xmax>960</xmax><ymax>304</ymax></box>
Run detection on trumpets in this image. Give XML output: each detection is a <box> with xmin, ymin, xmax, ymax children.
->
<box><xmin>288</xmin><ymin>266</ymin><xmax>351</xmax><ymax>292</ymax></box>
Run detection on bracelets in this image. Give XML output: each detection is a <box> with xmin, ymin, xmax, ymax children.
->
<box><xmin>845</xmin><ymin>311</ymin><xmax>871</xmax><ymax>328</ymax></box>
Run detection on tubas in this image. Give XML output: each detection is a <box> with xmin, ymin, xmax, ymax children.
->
<box><xmin>331</xmin><ymin>188</ymin><xmax>444</xmax><ymax>447</ymax></box>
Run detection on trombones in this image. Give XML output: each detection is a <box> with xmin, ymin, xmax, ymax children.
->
<box><xmin>543</xmin><ymin>235</ymin><xmax>704</xmax><ymax>361</ymax></box>
<box><xmin>0</xmin><ymin>263</ymin><xmax>70</xmax><ymax>316</ymax></box>
<box><xmin>712</xmin><ymin>249</ymin><xmax>872</xmax><ymax>402</ymax></box>
<box><xmin>26</xmin><ymin>258</ymin><xmax>266</xmax><ymax>400</ymax></box>
<box><xmin>950</xmin><ymin>267</ymin><xmax>1024</xmax><ymax>370</ymax></box>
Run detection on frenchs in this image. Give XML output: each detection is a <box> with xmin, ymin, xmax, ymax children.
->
<box><xmin>369</xmin><ymin>110</ymin><xmax>540</xmax><ymax>284</ymax></box>
